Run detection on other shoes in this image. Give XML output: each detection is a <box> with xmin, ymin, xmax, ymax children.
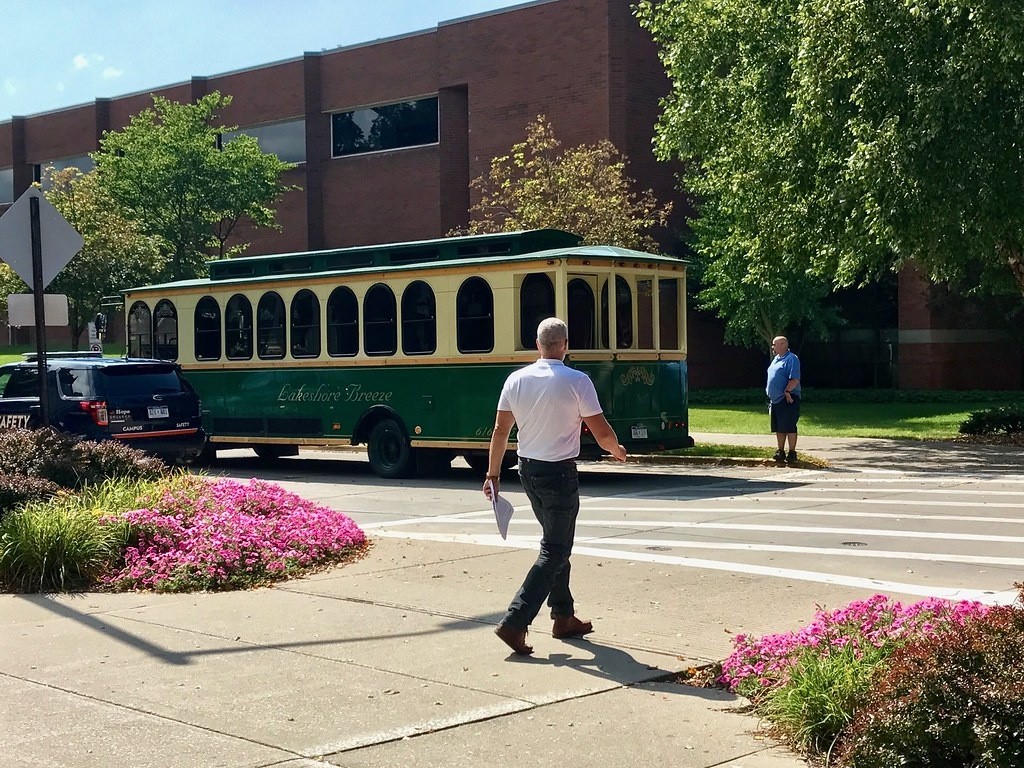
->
<box><xmin>773</xmin><ymin>449</ymin><xmax>786</xmax><ymax>460</ymax></box>
<box><xmin>786</xmin><ymin>450</ymin><xmax>797</xmax><ymax>462</ymax></box>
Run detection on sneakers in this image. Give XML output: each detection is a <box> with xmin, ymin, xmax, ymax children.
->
<box><xmin>494</xmin><ymin>622</ymin><xmax>533</xmax><ymax>655</ymax></box>
<box><xmin>552</xmin><ymin>615</ymin><xmax>594</xmax><ymax>638</ymax></box>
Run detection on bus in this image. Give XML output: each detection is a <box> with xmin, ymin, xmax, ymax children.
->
<box><xmin>94</xmin><ymin>228</ymin><xmax>696</xmax><ymax>479</ymax></box>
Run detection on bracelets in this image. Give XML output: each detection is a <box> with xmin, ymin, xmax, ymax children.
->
<box><xmin>485</xmin><ymin>473</ymin><xmax>500</xmax><ymax>480</ymax></box>
<box><xmin>786</xmin><ymin>390</ymin><xmax>790</xmax><ymax>393</ymax></box>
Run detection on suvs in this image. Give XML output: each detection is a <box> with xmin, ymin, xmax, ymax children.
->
<box><xmin>0</xmin><ymin>357</ymin><xmax>204</xmax><ymax>465</ymax></box>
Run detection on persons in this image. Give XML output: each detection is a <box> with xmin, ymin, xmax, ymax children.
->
<box><xmin>483</xmin><ymin>316</ymin><xmax>627</xmax><ymax>656</ymax></box>
<box><xmin>765</xmin><ymin>336</ymin><xmax>802</xmax><ymax>462</ymax></box>
